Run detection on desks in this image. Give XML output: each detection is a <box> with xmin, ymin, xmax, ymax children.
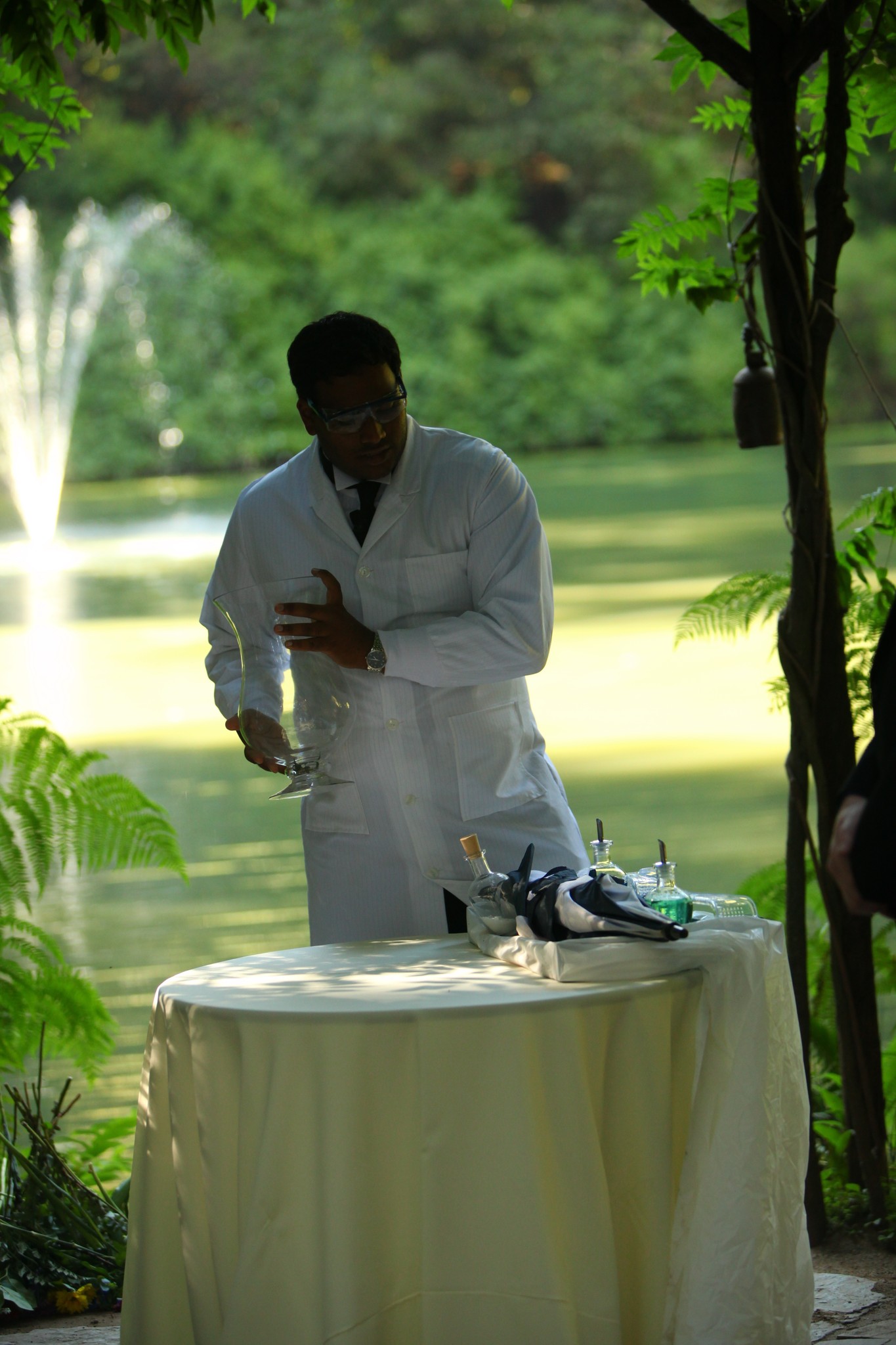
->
<box><xmin>120</xmin><ymin>935</ymin><xmax>816</xmax><ymax>1345</ymax></box>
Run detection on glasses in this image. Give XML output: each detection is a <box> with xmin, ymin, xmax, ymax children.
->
<box><xmin>306</xmin><ymin>374</ymin><xmax>407</xmax><ymax>431</ymax></box>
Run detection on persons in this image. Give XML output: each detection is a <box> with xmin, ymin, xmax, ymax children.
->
<box><xmin>199</xmin><ymin>310</ymin><xmax>591</xmax><ymax>946</ymax></box>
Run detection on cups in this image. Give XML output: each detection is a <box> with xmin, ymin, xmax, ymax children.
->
<box><xmin>210</xmin><ymin>574</ymin><xmax>361</xmax><ymax>801</ymax></box>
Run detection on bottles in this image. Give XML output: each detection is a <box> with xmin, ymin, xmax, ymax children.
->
<box><xmin>459</xmin><ymin>827</ymin><xmax>764</xmax><ymax>938</ymax></box>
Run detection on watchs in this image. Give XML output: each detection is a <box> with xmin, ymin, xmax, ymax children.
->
<box><xmin>365</xmin><ymin>629</ymin><xmax>388</xmax><ymax>676</ymax></box>
